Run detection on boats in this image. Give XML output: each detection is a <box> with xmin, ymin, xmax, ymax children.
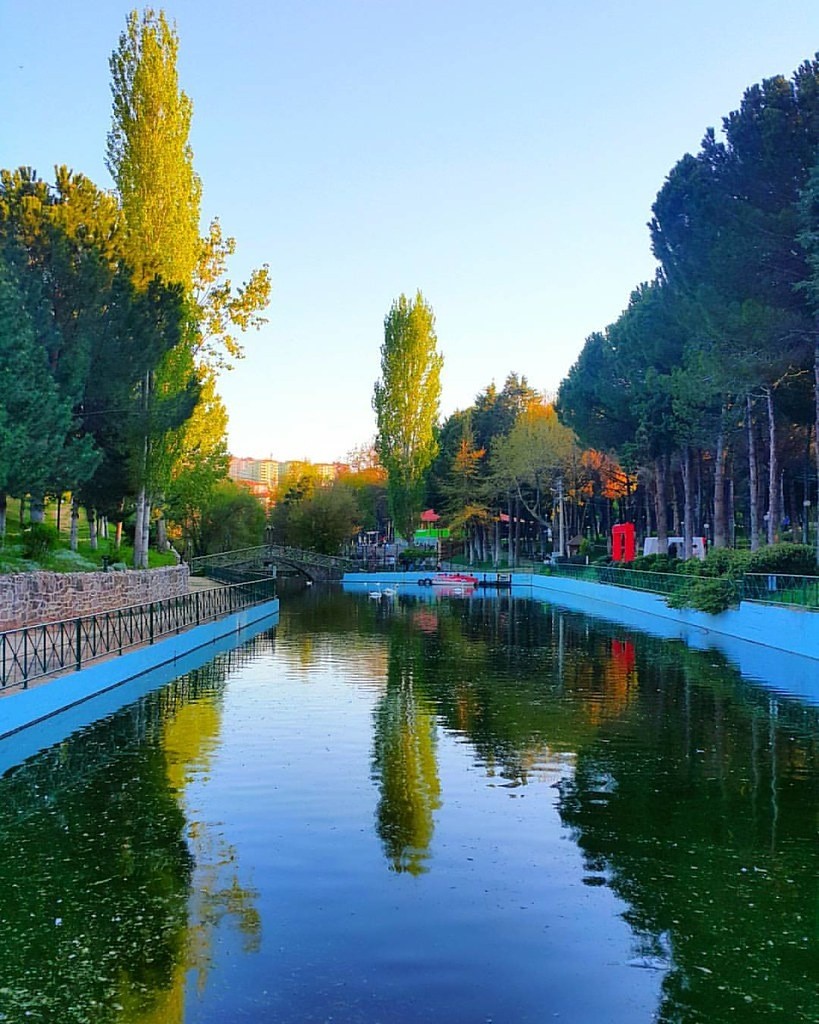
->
<box><xmin>429</xmin><ymin>573</ymin><xmax>480</xmax><ymax>591</ymax></box>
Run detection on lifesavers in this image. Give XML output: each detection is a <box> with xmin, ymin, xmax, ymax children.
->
<box><xmin>418</xmin><ymin>579</ymin><xmax>424</xmax><ymax>585</ymax></box>
<box><xmin>424</xmin><ymin>578</ymin><xmax>432</xmax><ymax>584</ymax></box>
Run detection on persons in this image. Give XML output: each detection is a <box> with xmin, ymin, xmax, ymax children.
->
<box><xmin>669</xmin><ymin>542</ymin><xmax>677</xmax><ymax>558</ymax></box>
<box><xmin>679</xmin><ymin>542</ymin><xmax>698</xmax><ymax>557</ymax></box>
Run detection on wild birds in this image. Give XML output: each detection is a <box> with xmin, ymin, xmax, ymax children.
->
<box><xmin>369</xmin><ymin>589</ymin><xmax>381</xmax><ymax>596</ymax></box>
<box><xmin>385</xmin><ymin>584</ymin><xmax>398</xmax><ymax>595</ymax></box>
